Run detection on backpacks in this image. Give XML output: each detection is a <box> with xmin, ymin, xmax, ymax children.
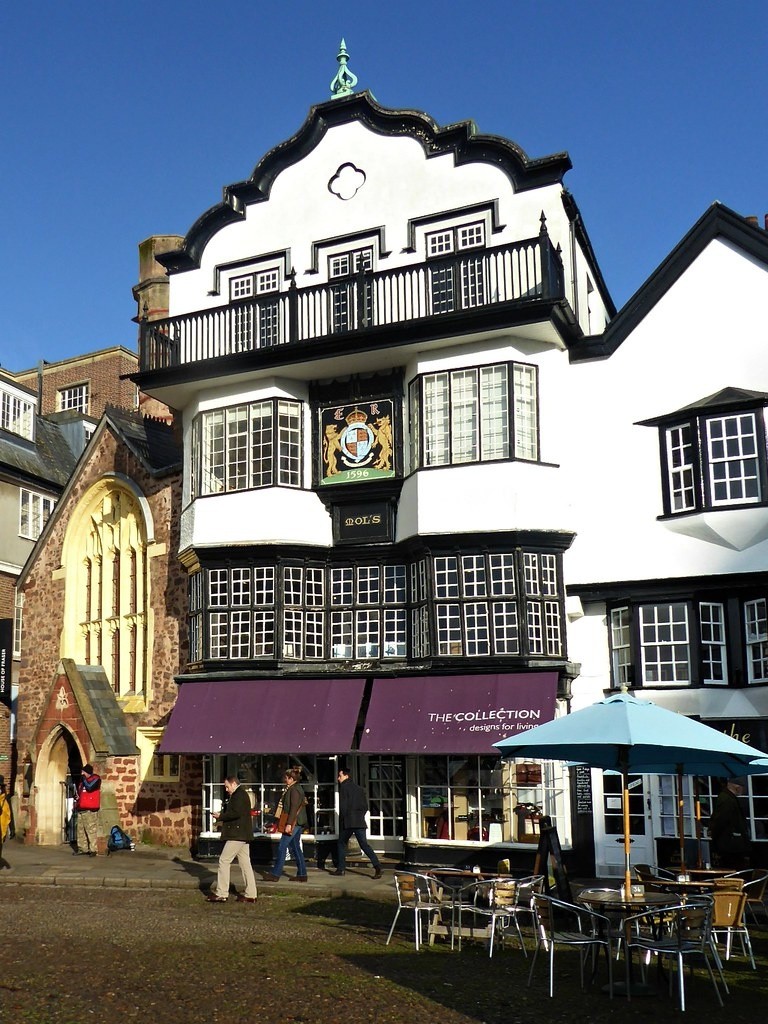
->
<box><xmin>107</xmin><ymin>825</ymin><xmax>130</xmax><ymax>851</ymax></box>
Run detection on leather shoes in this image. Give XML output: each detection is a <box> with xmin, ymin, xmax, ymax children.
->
<box><xmin>207</xmin><ymin>893</ymin><xmax>227</xmax><ymax>902</ymax></box>
<box><xmin>235</xmin><ymin>895</ymin><xmax>258</xmax><ymax>904</ymax></box>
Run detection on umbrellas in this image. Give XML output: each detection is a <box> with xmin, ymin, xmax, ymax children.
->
<box><xmin>491</xmin><ymin>682</ymin><xmax>768</xmax><ymax>896</ymax></box>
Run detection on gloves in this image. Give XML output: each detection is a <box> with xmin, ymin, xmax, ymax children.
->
<box><xmin>80</xmin><ymin>774</ymin><xmax>86</xmax><ymax>780</ymax></box>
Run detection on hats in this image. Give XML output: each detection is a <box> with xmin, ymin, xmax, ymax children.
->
<box><xmin>82</xmin><ymin>763</ymin><xmax>93</xmax><ymax>776</ymax></box>
<box><xmin>727</xmin><ymin>775</ymin><xmax>745</xmax><ymax>786</ymax></box>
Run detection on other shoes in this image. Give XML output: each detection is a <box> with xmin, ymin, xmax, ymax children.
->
<box><xmin>263</xmin><ymin>873</ymin><xmax>279</xmax><ymax>881</ymax></box>
<box><xmin>288</xmin><ymin>875</ymin><xmax>308</xmax><ymax>882</ymax></box>
<box><xmin>329</xmin><ymin>868</ymin><xmax>345</xmax><ymax>876</ymax></box>
<box><xmin>89</xmin><ymin>851</ymin><xmax>97</xmax><ymax>857</ymax></box>
<box><xmin>72</xmin><ymin>849</ymin><xmax>89</xmax><ymax>855</ymax></box>
<box><xmin>371</xmin><ymin>867</ymin><xmax>384</xmax><ymax>879</ymax></box>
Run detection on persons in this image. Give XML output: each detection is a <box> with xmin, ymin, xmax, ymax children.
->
<box><xmin>206</xmin><ymin>775</ymin><xmax>257</xmax><ymax>903</ymax></box>
<box><xmin>329</xmin><ymin>768</ymin><xmax>384</xmax><ymax>879</ymax></box>
<box><xmin>73</xmin><ymin>764</ymin><xmax>101</xmax><ymax>857</ymax></box>
<box><xmin>709</xmin><ymin>777</ymin><xmax>751</xmax><ymax>868</ymax></box>
<box><xmin>261</xmin><ymin>765</ymin><xmax>308</xmax><ymax>882</ymax></box>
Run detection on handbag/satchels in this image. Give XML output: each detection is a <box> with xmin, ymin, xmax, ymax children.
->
<box><xmin>278</xmin><ymin>813</ymin><xmax>296</xmax><ymax>835</ymax></box>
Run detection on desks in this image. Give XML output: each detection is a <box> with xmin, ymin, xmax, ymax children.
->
<box><xmin>421</xmin><ymin>806</ymin><xmax>447</xmax><ymax>837</ymax></box>
<box><xmin>665</xmin><ymin>866</ymin><xmax>736</xmax><ymax>938</ymax></box>
<box><xmin>577</xmin><ymin>891</ymin><xmax>680</xmax><ymax>997</ymax></box>
<box><xmin>417</xmin><ymin>869</ymin><xmax>513</xmax><ymax>951</ymax></box>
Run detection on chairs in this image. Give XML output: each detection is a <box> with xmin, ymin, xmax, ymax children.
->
<box><xmin>385</xmin><ymin>863</ymin><xmax>768</xmax><ymax>1012</ymax></box>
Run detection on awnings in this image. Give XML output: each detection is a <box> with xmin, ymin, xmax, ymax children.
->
<box><xmin>360</xmin><ymin>672</ymin><xmax>559</xmax><ymax>753</ymax></box>
<box><xmin>153</xmin><ymin>679</ymin><xmax>368</xmax><ymax>754</ymax></box>
